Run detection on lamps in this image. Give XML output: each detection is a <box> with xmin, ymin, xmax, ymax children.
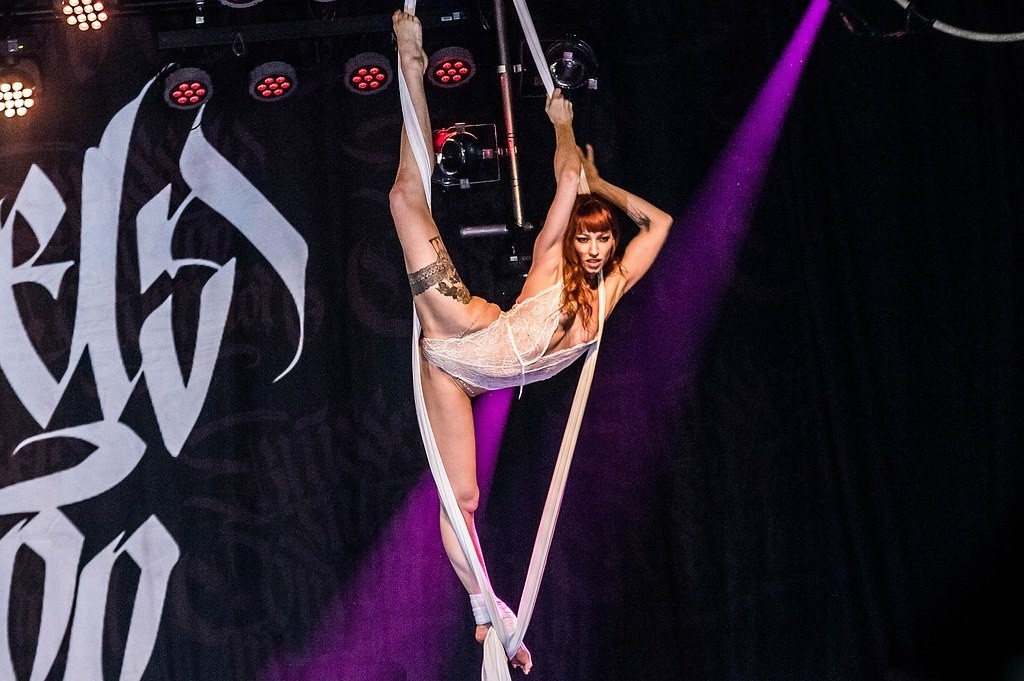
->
<box><xmin>160</xmin><ymin>47</ymin><xmax>214</xmax><ymax>109</ymax></box>
<box><xmin>247</xmin><ymin>39</ymin><xmax>300</xmax><ymax>102</ymax></box>
<box><xmin>424</xmin><ymin>27</ymin><xmax>477</xmax><ymax>88</ymax></box>
<box><xmin>339</xmin><ymin>31</ymin><xmax>394</xmax><ymax>96</ymax></box>
<box><xmin>546</xmin><ymin>31</ymin><xmax>598</xmax><ymax>100</ymax></box>
<box><xmin>55</xmin><ymin>0</ymin><xmax>117</xmax><ymax>37</ymax></box>
<box><xmin>430</xmin><ymin>126</ymin><xmax>484</xmax><ymax>186</ymax></box>
<box><xmin>0</xmin><ymin>56</ymin><xmax>46</xmax><ymax>123</ymax></box>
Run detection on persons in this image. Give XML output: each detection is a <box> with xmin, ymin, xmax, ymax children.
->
<box><xmin>384</xmin><ymin>8</ymin><xmax>675</xmax><ymax>672</ymax></box>
